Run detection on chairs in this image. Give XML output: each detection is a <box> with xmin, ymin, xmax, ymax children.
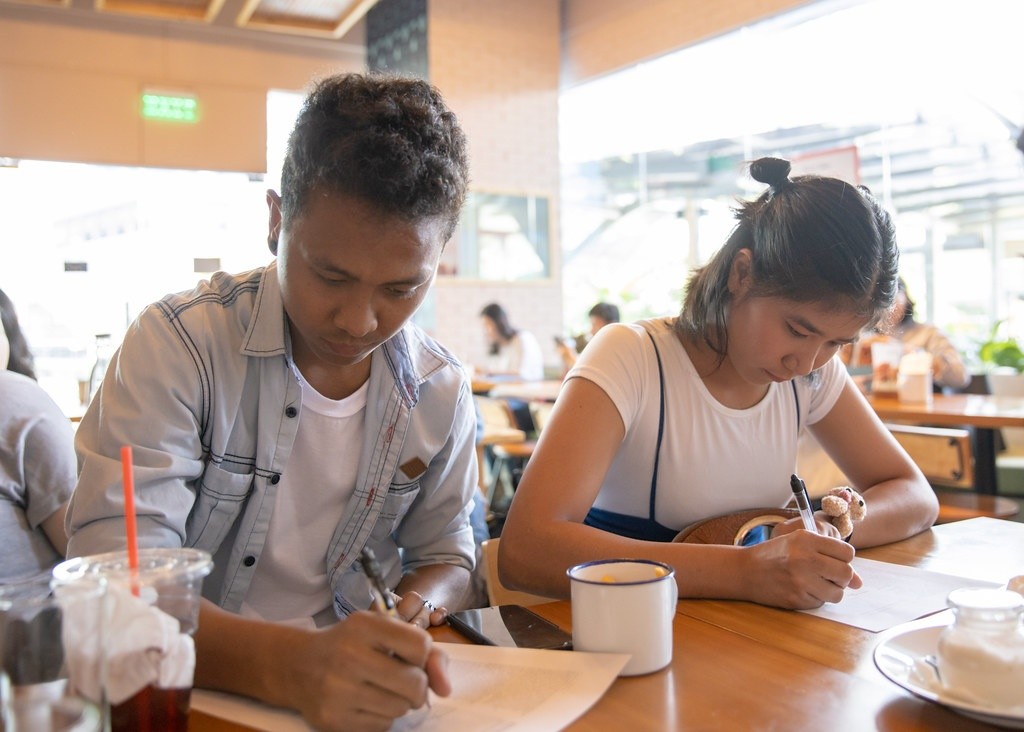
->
<box><xmin>473</xmin><ymin>395</ymin><xmax>538</xmax><ymax>513</ymax></box>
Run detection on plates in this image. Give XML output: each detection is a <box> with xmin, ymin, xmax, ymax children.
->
<box><xmin>872</xmin><ymin>613</ymin><xmax>1024</xmax><ymax>730</ymax></box>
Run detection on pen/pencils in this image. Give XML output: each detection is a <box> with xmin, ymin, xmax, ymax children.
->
<box><xmin>360</xmin><ymin>547</ymin><xmax>430</xmax><ymax>710</ymax></box>
<box><xmin>791</xmin><ymin>474</ymin><xmax>818</xmax><ymax>534</ymax></box>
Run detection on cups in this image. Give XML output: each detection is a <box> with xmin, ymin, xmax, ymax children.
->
<box><xmin>51</xmin><ymin>549</ymin><xmax>213</xmax><ymax>732</ymax></box>
<box><xmin>897</xmin><ymin>372</ymin><xmax>932</xmax><ymax>402</ymax></box>
<box><xmin>0</xmin><ymin>575</ymin><xmax>112</xmax><ymax>732</ymax></box>
<box><xmin>871</xmin><ymin>343</ymin><xmax>902</xmax><ymax>398</ymax></box>
<box><xmin>566</xmin><ymin>557</ymin><xmax>679</xmax><ymax>676</ymax></box>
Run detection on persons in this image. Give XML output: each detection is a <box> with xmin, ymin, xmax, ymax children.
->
<box><xmin>0</xmin><ymin>288</ymin><xmax>78</xmax><ymax>683</ymax></box>
<box><xmin>476</xmin><ymin>300</ymin><xmax>542</xmax><ymax>383</ymax></box>
<box><xmin>857</xmin><ymin>277</ymin><xmax>968</xmax><ymax>399</ymax></box>
<box><xmin>497</xmin><ymin>158</ymin><xmax>939</xmax><ymax>613</ymax></box>
<box><xmin>65</xmin><ymin>67</ymin><xmax>479</xmax><ymax>732</ymax></box>
<box><xmin>558</xmin><ymin>299</ymin><xmax>620</xmax><ymax>378</ymax></box>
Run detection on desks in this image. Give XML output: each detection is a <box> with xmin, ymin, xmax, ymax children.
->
<box><xmin>0</xmin><ymin>517</ymin><xmax>1024</xmax><ymax>732</ymax></box>
<box><xmin>862</xmin><ymin>390</ymin><xmax>1024</xmax><ymax>497</ymax></box>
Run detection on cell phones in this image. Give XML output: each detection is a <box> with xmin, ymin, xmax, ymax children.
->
<box><xmin>448</xmin><ymin>604</ymin><xmax>573</xmax><ymax>651</ymax></box>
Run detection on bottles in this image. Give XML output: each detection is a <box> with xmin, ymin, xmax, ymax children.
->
<box><xmin>937</xmin><ymin>587</ymin><xmax>1024</xmax><ymax>713</ymax></box>
